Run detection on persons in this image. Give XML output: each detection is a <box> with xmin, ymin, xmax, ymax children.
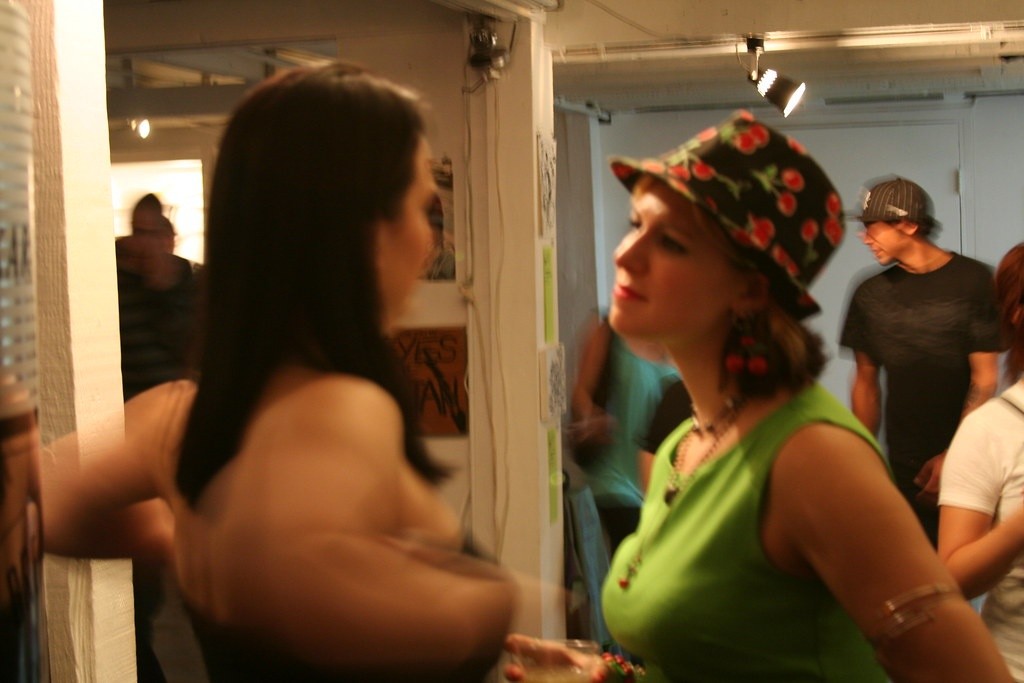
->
<box><xmin>39</xmin><ymin>47</ymin><xmax>522</xmax><ymax>683</ymax></box>
<box><xmin>936</xmin><ymin>238</ymin><xmax>1024</xmax><ymax>683</ymax></box>
<box><xmin>565</xmin><ymin>299</ymin><xmax>697</xmax><ymax>566</ymax></box>
<box><xmin>500</xmin><ymin>106</ymin><xmax>1024</xmax><ymax>682</ymax></box>
<box><xmin>115</xmin><ymin>189</ymin><xmax>203</xmax><ymax>403</ymax></box>
<box><xmin>836</xmin><ymin>174</ymin><xmax>1001</xmax><ymax>551</ymax></box>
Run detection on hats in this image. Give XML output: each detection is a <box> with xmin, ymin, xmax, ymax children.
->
<box><xmin>609</xmin><ymin>110</ymin><xmax>847</xmax><ymax>319</ymax></box>
<box><xmin>855</xmin><ymin>175</ymin><xmax>940</xmax><ymax>228</ymax></box>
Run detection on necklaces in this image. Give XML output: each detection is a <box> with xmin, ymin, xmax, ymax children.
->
<box><xmin>620</xmin><ymin>393</ymin><xmax>751</xmax><ymax>590</ymax></box>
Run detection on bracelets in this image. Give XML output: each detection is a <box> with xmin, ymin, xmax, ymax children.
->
<box><xmin>603</xmin><ymin>650</ymin><xmax>646</xmax><ymax>683</ymax></box>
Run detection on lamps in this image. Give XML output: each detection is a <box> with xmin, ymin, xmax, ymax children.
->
<box><xmin>124</xmin><ymin>117</ymin><xmax>151</xmax><ymax>139</ymax></box>
<box><xmin>745</xmin><ymin>37</ymin><xmax>807</xmax><ymax>118</ymax></box>
